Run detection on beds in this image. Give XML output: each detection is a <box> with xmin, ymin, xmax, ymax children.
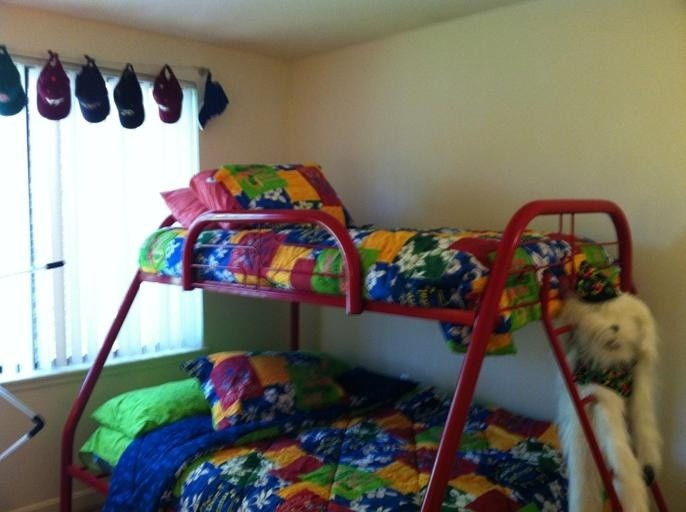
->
<box><xmin>59</xmin><ymin>196</ymin><xmax>668</xmax><ymax>512</ymax></box>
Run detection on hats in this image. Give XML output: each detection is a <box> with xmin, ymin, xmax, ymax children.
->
<box><xmin>35</xmin><ymin>51</ymin><xmax>71</xmax><ymax>121</ymax></box>
<box><xmin>72</xmin><ymin>58</ymin><xmax>110</xmax><ymax>123</ymax></box>
<box><xmin>0</xmin><ymin>43</ymin><xmax>25</xmax><ymax>116</ymax></box>
<box><xmin>151</xmin><ymin>63</ymin><xmax>183</xmax><ymax>124</ymax></box>
<box><xmin>111</xmin><ymin>62</ymin><xmax>145</xmax><ymax>129</ymax></box>
<box><xmin>196</xmin><ymin>69</ymin><xmax>229</xmax><ymax>131</ymax></box>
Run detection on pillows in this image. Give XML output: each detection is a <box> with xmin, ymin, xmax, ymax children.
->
<box><xmin>160</xmin><ymin>162</ymin><xmax>356</xmax><ymax>230</ymax></box>
<box><xmin>77</xmin><ymin>348</ymin><xmax>352</xmax><ymax>481</ymax></box>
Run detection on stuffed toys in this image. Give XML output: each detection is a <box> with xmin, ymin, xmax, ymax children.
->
<box><xmin>551</xmin><ymin>260</ymin><xmax>665</xmax><ymax>510</ymax></box>
